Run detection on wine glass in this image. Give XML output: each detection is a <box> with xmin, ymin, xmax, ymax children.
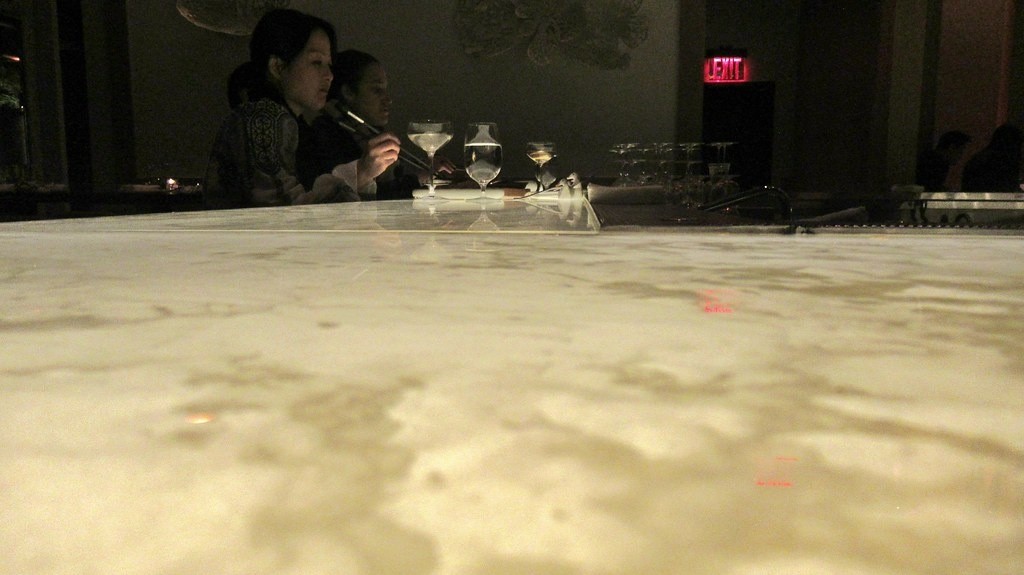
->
<box><xmin>526</xmin><ymin>142</ymin><xmax>557</xmax><ymax>195</ymax></box>
<box><xmin>406</xmin><ymin>120</ymin><xmax>453</xmax><ymax>198</ymax></box>
<box><xmin>610</xmin><ymin>141</ymin><xmax>740</xmax><ymax>190</ymax></box>
<box><xmin>465</xmin><ymin>201</ymin><xmax>500</xmax><ymax>252</ymax></box>
<box><xmin>464</xmin><ymin>123</ymin><xmax>502</xmax><ymax>199</ymax></box>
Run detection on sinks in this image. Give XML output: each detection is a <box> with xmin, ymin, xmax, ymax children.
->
<box><xmin>921</xmin><ymin>193</ymin><xmax>1024</xmax><ymax>225</ymax></box>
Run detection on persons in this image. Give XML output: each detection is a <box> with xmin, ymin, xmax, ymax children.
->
<box><xmin>201</xmin><ymin>8</ymin><xmax>457</xmax><ymax>211</ymax></box>
<box><xmin>930</xmin><ymin>124</ymin><xmax>1024</xmax><ymax>193</ymax></box>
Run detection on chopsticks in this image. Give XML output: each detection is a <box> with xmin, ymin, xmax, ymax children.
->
<box><xmin>339</xmin><ymin>111</ymin><xmax>428</xmax><ymax>170</ymax></box>
